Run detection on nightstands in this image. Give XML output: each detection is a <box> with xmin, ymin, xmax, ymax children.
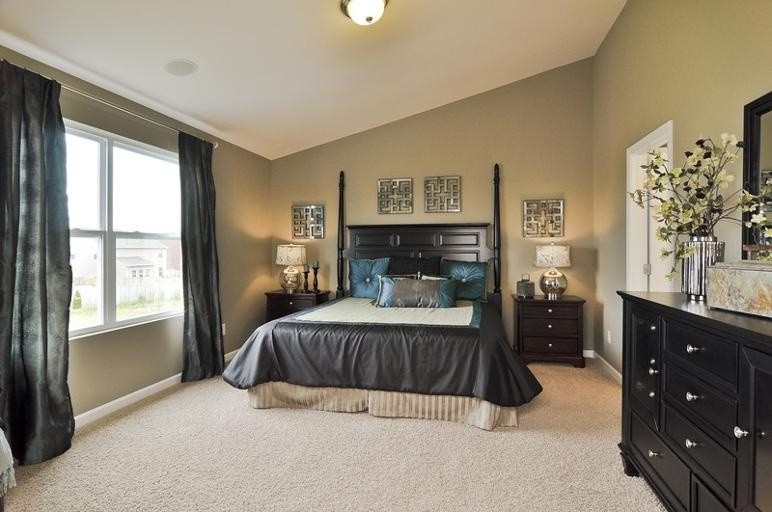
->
<box><xmin>509</xmin><ymin>294</ymin><xmax>587</xmax><ymax>369</ymax></box>
<box><xmin>263</xmin><ymin>289</ymin><xmax>331</xmax><ymax>323</ymax></box>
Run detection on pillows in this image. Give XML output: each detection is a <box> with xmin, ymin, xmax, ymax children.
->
<box><xmin>373</xmin><ymin>273</ymin><xmax>456</xmax><ymax>308</ymax></box>
<box><xmin>438</xmin><ymin>257</ymin><xmax>490</xmax><ymax>304</ymax></box>
<box><xmin>347</xmin><ymin>257</ymin><xmax>392</xmax><ymax>299</ymax></box>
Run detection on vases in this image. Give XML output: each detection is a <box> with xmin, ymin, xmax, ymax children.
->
<box><xmin>676</xmin><ymin>233</ymin><xmax>726</xmax><ymax>302</ymax></box>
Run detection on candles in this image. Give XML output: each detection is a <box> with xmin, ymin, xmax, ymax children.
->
<box><xmin>303</xmin><ymin>263</ymin><xmax>309</xmax><ymax>273</ymax></box>
<box><xmin>312</xmin><ymin>259</ymin><xmax>319</xmax><ymax>266</ymax></box>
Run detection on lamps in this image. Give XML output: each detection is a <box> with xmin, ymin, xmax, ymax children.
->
<box><xmin>533</xmin><ymin>242</ymin><xmax>572</xmax><ymax>302</ymax></box>
<box><xmin>339</xmin><ymin>0</ymin><xmax>390</xmax><ymax>27</ymax></box>
<box><xmin>274</xmin><ymin>242</ymin><xmax>307</xmax><ymax>294</ymax></box>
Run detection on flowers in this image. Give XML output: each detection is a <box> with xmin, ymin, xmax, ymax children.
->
<box><xmin>625</xmin><ymin>132</ymin><xmax>772</xmax><ymax>284</ymax></box>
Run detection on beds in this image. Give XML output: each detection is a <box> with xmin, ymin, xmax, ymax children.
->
<box><xmin>220</xmin><ymin>163</ymin><xmax>543</xmax><ymax>432</ymax></box>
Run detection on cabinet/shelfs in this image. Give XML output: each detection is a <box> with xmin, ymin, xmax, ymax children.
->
<box><xmin>614</xmin><ymin>287</ymin><xmax>772</xmax><ymax>512</ymax></box>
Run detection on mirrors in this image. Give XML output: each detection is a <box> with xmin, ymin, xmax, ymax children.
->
<box><xmin>741</xmin><ymin>90</ymin><xmax>772</xmax><ymax>261</ymax></box>
